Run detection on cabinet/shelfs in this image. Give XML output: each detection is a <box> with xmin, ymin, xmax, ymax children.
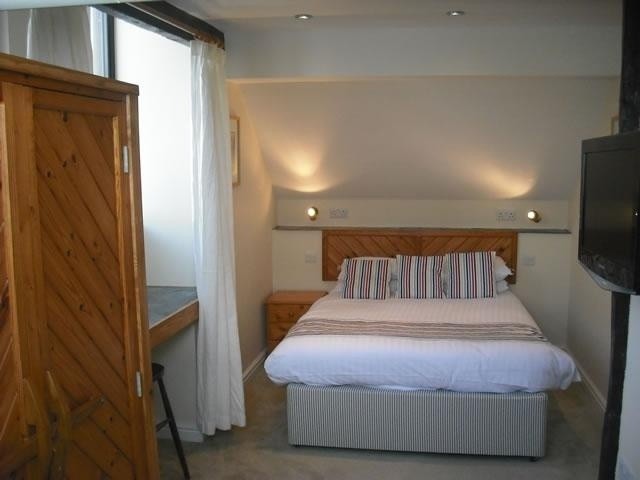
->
<box><xmin>0</xmin><ymin>49</ymin><xmax>167</xmax><ymax>478</ymax></box>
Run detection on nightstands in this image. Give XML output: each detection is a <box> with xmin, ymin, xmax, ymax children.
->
<box><xmin>263</xmin><ymin>289</ymin><xmax>326</xmax><ymax>357</ymax></box>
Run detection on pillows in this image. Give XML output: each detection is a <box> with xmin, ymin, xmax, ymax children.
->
<box><xmin>498</xmin><ymin>282</ymin><xmax>509</xmax><ymax>292</ymax></box>
<box><xmin>394</xmin><ymin>253</ymin><xmax>446</xmax><ymax>300</ymax></box>
<box><xmin>446</xmin><ymin>250</ymin><xmax>498</xmax><ymax>300</ymax></box>
<box><xmin>338</xmin><ymin>257</ymin><xmax>391</xmax><ymax>300</ymax></box>
<box><xmin>496</xmin><ymin>256</ymin><xmax>512</xmax><ymax>281</ymax></box>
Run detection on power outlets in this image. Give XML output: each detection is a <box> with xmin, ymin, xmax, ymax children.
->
<box><xmin>329</xmin><ymin>206</ymin><xmax>350</xmax><ymax>219</ymax></box>
<box><xmin>494</xmin><ymin>209</ymin><xmax>518</xmax><ymax>223</ymax></box>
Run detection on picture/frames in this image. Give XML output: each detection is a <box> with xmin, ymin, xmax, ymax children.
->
<box><xmin>229</xmin><ymin>113</ymin><xmax>241</xmax><ymax>189</ymax></box>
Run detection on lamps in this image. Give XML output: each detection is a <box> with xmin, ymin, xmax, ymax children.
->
<box><xmin>527</xmin><ymin>209</ymin><xmax>541</xmax><ymax>223</ymax></box>
<box><xmin>307</xmin><ymin>206</ymin><xmax>319</xmax><ymax>222</ymax></box>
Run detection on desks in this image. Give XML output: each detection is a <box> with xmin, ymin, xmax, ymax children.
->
<box><xmin>147</xmin><ymin>281</ymin><xmax>198</xmax><ymax>347</ymax></box>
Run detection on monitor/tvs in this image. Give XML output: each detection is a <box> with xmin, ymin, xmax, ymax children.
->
<box><xmin>576</xmin><ymin>135</ymin><xmax>639</xmax><ymax>295</ymax></box>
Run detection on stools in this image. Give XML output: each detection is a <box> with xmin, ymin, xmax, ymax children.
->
<box><xmin>152</xmin><ymin>362</ymin><xmax>192</xmax><ymax>480</ymax></box>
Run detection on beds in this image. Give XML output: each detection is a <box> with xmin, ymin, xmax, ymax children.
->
<box><xmin>264</xmin><ymin>227</ymin><xmax>582</xmax><ymax>462</ymax></box>
